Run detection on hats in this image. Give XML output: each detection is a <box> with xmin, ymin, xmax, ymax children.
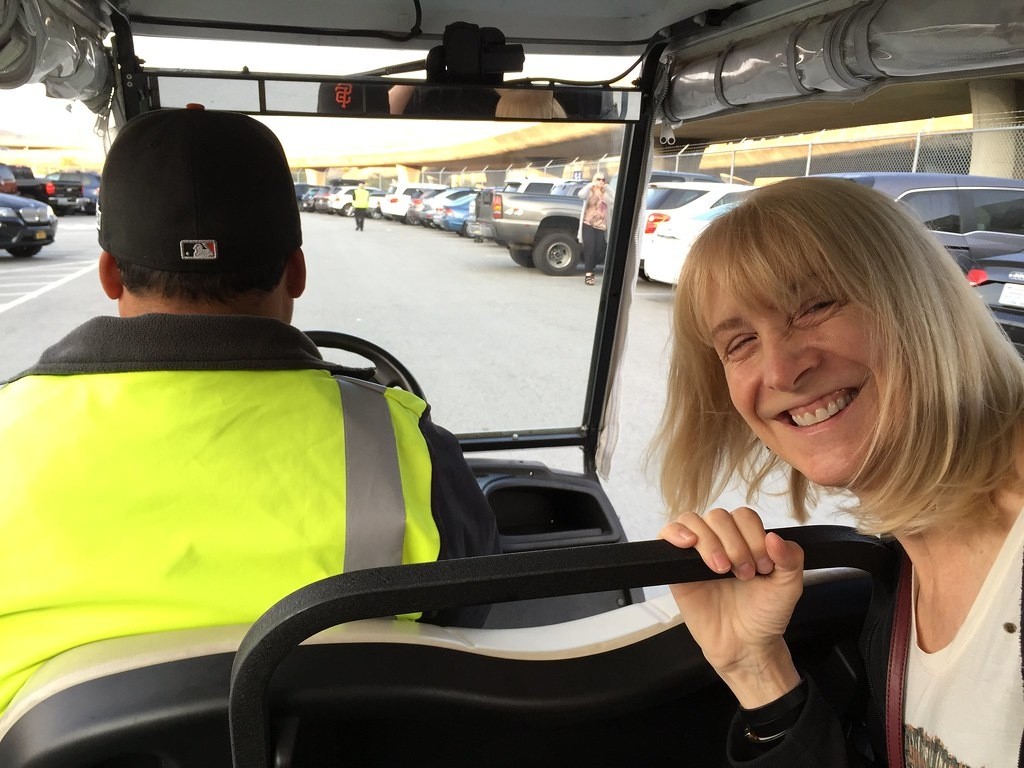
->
<box><xmin>95</xmin><ymin>101</ymin><xmax>303</xmax><ymax>273</ymax></box>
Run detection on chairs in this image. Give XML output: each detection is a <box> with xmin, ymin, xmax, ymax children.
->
<box><xmin>0</xmin><ymin>557</ymin><xmax>913</xmax><ymax>768</ymax></box>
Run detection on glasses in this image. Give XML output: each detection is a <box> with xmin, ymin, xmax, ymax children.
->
<box><xmin>596</xmin><ymin>177</ymin><xmax>606</xmax><ymax>182</ymax></box>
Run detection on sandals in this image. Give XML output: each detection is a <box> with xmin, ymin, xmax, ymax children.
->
<box><xmin>585</xmin><ymin>272</ymin><xmax>595</xmax><ymax>286</ymax></box>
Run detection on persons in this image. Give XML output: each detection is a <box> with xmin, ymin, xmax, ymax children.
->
<box><xmin>660</xmin><ymin>177</ymin><xmax>1024</xmax><ymax>768</ymax></box>
<box><xmin>578</xmin><ymin>171</ymin><xmax>615</xmax><ymax>285</ymax></box>
<box><xmin>0</xmin><ymin>100</ymin><xmax>502</xmax><ymax>720</ymax></box>
<box><xmin>317</xmin><ymin>80</ymin><xmax>416</xmax><ymax>115</ymax></box>
<box><xmin>351</xmin><ymin>181</ymin><xmax>369</xmax><ymax>230</ymax></box>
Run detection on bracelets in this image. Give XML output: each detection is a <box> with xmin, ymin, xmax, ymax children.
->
<box><xmin>741</xmin><ymin>724</ymin><xmax>789</xmax><ymax>743</ymax></box>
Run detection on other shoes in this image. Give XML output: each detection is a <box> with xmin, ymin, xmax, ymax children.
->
<box><xmin>356</xmin><ymin>225</ymin><xmax>363</xmax><ymax>231</ymax></box>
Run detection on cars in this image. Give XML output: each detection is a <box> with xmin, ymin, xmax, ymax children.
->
<box><xmin>0</xmin><ymin>192</ymin><xmax>58</xmax><ymax>256</ymax></box>
<box><xmin>46</xmin><ymin>172</ymin><xmax>101</xmax><ymax>215</ymax></box>
<box><xmin>638</xmin><ymin>181</ymin><xmax>760</xmax><ymax>285</ymax></box>
<box><xmin>294</xmin><ymin>177</ymin><xmax>592</xmax><ymax>246</ymax></box>
<box><xmin>965</xmin><ymin>254</ymin><xmax>1024</xmax><ymax>359</ymax></box>
<box><xmin>817</xmin><ymin>172</ymin><xmax>1024</xmax><ymax>272</ymax></box>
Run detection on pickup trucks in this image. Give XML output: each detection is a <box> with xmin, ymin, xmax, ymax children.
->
<box><xmin>8</xmin><ymin>165</ymin><xmax>84</xmax><ymax>217</ymax></box>
<box><xmin>466</xmin><ymin>170</ymin><xmax>724</xmax><ymax>276</ymax></box>
